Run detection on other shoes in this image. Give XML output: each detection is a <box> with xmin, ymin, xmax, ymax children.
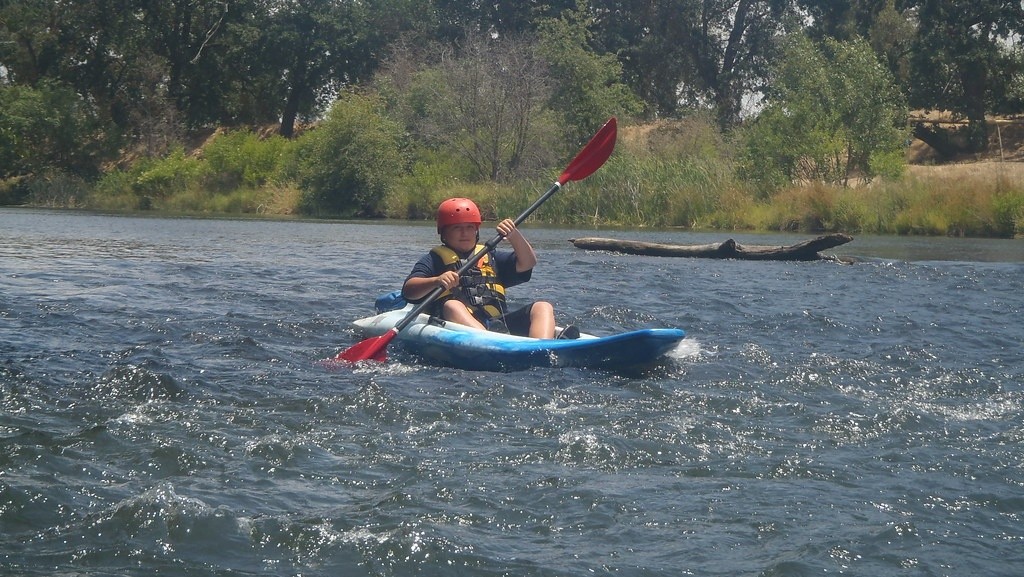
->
<box><xmin>555</xmin><ymin>323</ymin><xmax>583</xmax><ymax>341</ymax></box>
<box><xmin>487</xmin><ymin>317</ymin><xmax>510</xmax><ymax>336</ymax></box>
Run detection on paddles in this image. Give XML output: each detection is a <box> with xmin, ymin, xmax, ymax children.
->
<box><xmin>336</xmin><ymin>115</ymin><xmax>618</xmax><ymax>365</ymax></box>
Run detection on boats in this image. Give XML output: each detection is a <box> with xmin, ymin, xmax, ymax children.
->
<box><xmin>350</xmin><ymin>305</ymin><xmax>687</xmax><ymax>375</ymax></box>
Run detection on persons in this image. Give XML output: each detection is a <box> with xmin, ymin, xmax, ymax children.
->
<box><xmin>402</xmin><ymin>197</ymin><xmax>580</xmax><ymax>339</ymax></box>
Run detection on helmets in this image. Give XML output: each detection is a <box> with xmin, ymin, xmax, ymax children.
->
<box><xmin>435</xmin><ymin>197</ymin><xmax>482</xmax><ymax>234</ymax></box>
